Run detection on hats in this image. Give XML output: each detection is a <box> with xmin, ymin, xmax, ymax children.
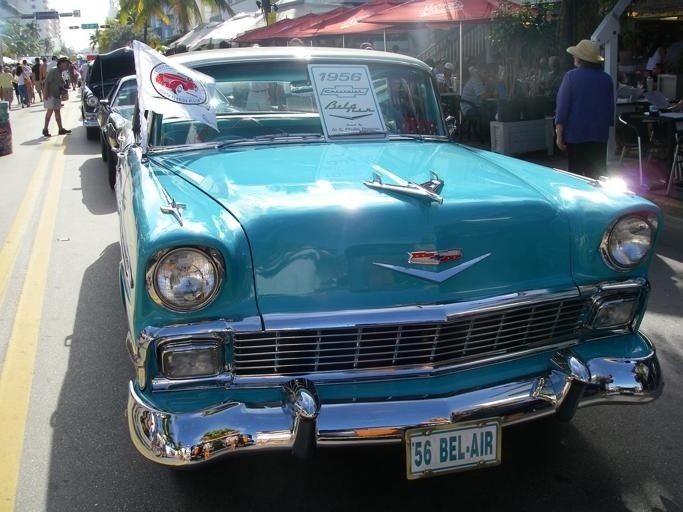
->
<box><xmin>57</xmin><ymin>57</ymin><xmax>72</xmax><ymax>64</ymax></box>
<box><xmin>566</xmin><ymin>40</ymin><xmax>604</xmax><ymax>64</ymax></box>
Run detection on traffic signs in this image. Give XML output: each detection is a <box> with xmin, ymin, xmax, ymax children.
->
<box><xmin>80</xmin><ymin>24</ymin><xmax>98</xmax><ymax>30</ymax></box>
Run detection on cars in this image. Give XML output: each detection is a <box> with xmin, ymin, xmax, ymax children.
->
<box><xmin>78</xmin><ymin>46</ymin><xmax>141</xmax><ymax>141</ymax></box>
<box><xmin>93</xmin><ymin>72</ymin><xmax>245</xmax><ymax>191</ymax></box>
<box><xmin>111</xmin><ymin>46</ymin><xmax>667</xmax><ymax>489</ymax></box>
<box><xmin>157</xmin><ymin>72</ymin><xmax>199</xmax><ymax>94</ymax></box>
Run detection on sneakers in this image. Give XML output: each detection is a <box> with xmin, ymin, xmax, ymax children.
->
<box><xmin>59</xmin><ymin>129</ymin><xmax>71</xmax><ymax>134</ymax></box>
<box><xmin>43</xmin><ymin>130</ymin><xmax>51</xmax><ymax>136</ymax></box>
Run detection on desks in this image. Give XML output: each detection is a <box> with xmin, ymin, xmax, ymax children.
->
<box><xmin>617</xmin><ymin>98</ymin><xmax>677</xmax><ymax>112</ymax></box>
<box><xmin>660</xmin><ymin>112</ymin><xmax>682</xmax><ymax>193</ymax></box>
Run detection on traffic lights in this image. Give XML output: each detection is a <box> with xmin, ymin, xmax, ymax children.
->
<box><xmin>69</xmin><ymin>26</ymin><xmax>79</xmax><ymax>30</ymax></box>
<box><xmin>100</xmin><ymin>25</ymin><xmax>110</xmax><ymax>29</ymax></box>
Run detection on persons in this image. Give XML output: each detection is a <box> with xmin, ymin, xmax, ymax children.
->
<box><xmin>460</xmin><ymin>52</ymin><xmax>683</xmax><ymax>150</ymax></box>
<box><xmin>177</xmin><ymin>39</ymin><xmax>552</xmax><ymax>124</ymax></box>
<box><xmin>0</xmin><ymin>53</ymin><xmax>98</xmax><ymax>137</ymax></box>
<box><xmin>554</xmin><ymin>40</ymin><xmax>613</xmax><ymax>180</ymax></box>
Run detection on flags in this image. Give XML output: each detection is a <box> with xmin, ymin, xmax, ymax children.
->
<box><xmin>132</xmin><ymin>39</ymin><xmax>219</xmax><ymax>131</ymax></box>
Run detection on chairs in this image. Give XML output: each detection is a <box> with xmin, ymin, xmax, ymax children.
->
<box><xmin>615</xmin><ymin>113</ymin><xmax>680</xmax><ymax>191</ymax></box>
<box><xmin>161</xmin><ymin>117</ymin><xmax>322</xmax><ymax>148</ymax></box>
<box><xmin>434</xmin><ymin>70</ymin><xmax>556</xmax><ymax>142</ymax></box>
